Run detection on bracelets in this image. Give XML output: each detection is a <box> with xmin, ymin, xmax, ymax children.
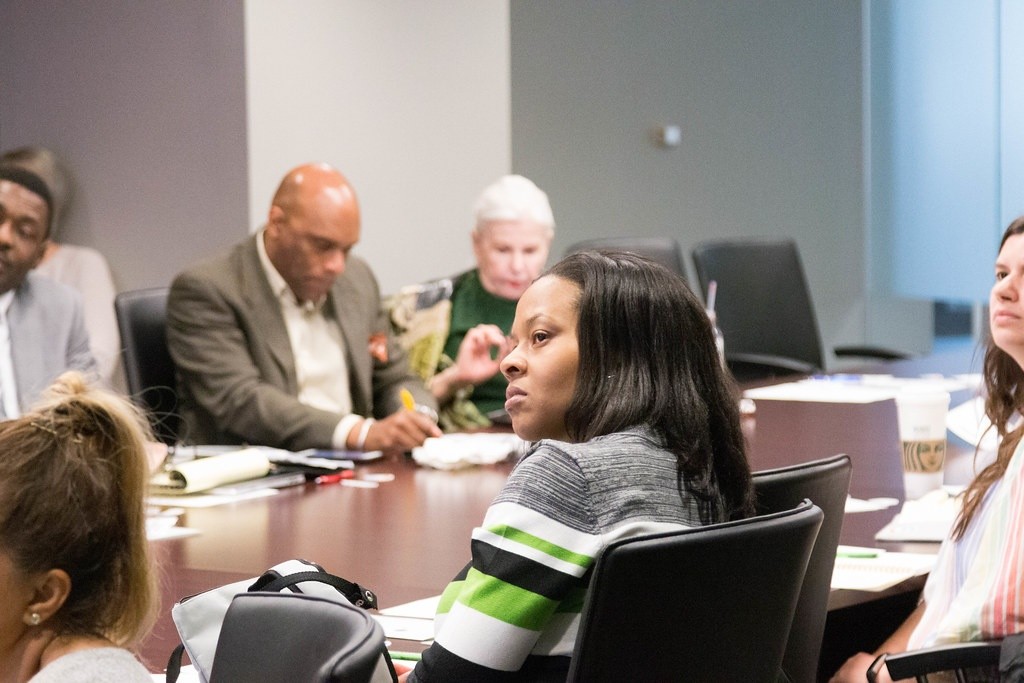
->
<box><xmin>357</xmin><ymin>416</ymin><xmax>376</xmax><ymax>452</ymax></box>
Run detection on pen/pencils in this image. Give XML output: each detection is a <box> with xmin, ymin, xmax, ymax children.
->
<box><xmin>810</xmin><ymin>373</ymin><xmax>862</xmax><ymax>380</ymax></box>
<box><xmin>400</xmin><ymin>388</ymin><xmax>417</xmax><ymax>411</ymax></box>
<box><xmin>706</xmin><ymin>280</ymin><xmax>718</xmax><ymax>311</ymax></box>
<box><xmin>389</xmin><ymin>652</ymin><xmax>421</xmax><ymax>661</ymax></box>
<box><xmin>835</xmin><ymin>552</ymin><xmax>877</xmax><ymax>559</ymax></box>
<box><xmin>316</xmin><ymin>470</ymin><xmax>354</xmax><ymax>484</ymax></box>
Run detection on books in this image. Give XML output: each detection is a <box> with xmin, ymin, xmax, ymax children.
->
<box><xmin>146</xmin><ymin>447</ymin><xmax>306</xmax><ymax>497</ymax></box>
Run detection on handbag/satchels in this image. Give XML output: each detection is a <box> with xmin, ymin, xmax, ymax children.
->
<box><xmin>165</xmin><ymin>559</ymin><xmax>399</xmax><ymax>683</ymax></box>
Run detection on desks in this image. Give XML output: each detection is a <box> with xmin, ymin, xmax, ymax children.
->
<box><xmin>128</xmin><ymin>365</ymin><xmax>1003</xmax><ymax>683</ymax></box>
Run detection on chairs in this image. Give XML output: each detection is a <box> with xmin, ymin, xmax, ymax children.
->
<box><xmin>208</xmin><ymin>590</ymin><xmax>386</xmax><ymax>683</ymax></box>
<box><xmin>750</xmin><ymin>454</ymin><xmax>853</xmax><ymax>683</ymax></box>
<box><xmin>562</xmin><ymin>235</ymin><xmax>688</xmax><ymax>291</ymax></box>
<box><xmin>561</xmin><ymin>499</ymin><xmax>824</xmax><ymax>683</ymax></box>
<box><xmin>693</xmin><ymin>236</ymin><xmax>911</xmax><ymax>387</ymax></box>
<box><xmin>114</xmin><ymin>287</ymin><xmax>182</xmax><ymax>448</ymax></box>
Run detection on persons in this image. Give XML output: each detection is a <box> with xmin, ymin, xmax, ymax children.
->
<box><xmin>425</xmin><ymin>174</ymin><xmax>556</xmax><ymax>429</ymax></box>
<box><xmin>827</xmin><ymin>214</ymin><xmax>1024</xmax><ymax>683</ymax></box>
<box><xmin>391</xmin><ymin>248</ymin><xmax>755</xmax><ymax>683</ymax></box>
<box><xmin>0</xmin><ymin>371</ymin><xmax>160</xmax><ymax>683</ymax></box>
<box><xmin>0</xmin><ymin>145</ymin><xmax>128</xmax><ymax>420</ymax></box>
<box><xmin>165</xmin><ymin>163</ymin><xmax>443</xmax><ymax>463</ymax></box>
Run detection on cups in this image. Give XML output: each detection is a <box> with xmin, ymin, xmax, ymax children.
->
<box><xmin>879</xmin><ymin>380</ymin><xmax>951</xmax><ymax>501</ymax></box>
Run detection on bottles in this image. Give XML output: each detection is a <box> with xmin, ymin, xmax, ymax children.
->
<box><xmin>704</xmin><ymin>308</ymin><xmax>725</xmax><ymax>378</ymax></box>
